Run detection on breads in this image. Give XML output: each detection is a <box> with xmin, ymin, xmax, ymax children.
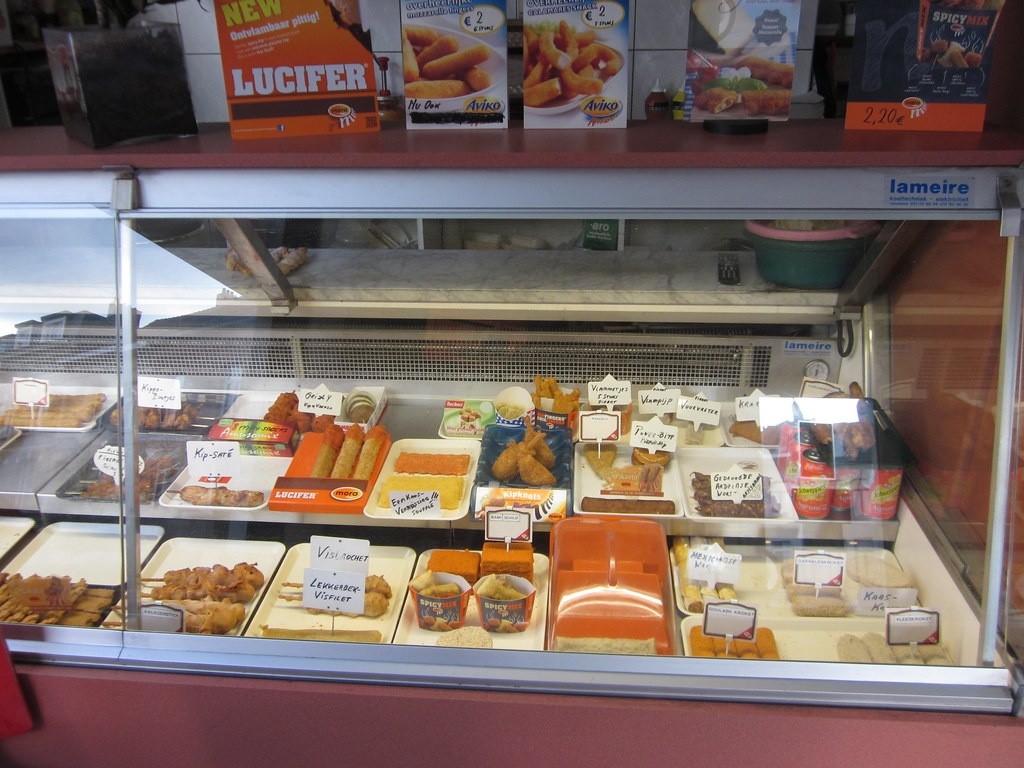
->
<box><xmin>783</xmin><ymin>567</ymin><xmax>953</xmax><ymax>666</ymax></box>
<box><xmin>693</xmin><ymin>56</ymin><xmax>795</xmax><ymax>115</ymax></box>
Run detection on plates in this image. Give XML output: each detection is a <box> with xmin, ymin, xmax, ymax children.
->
<box><xmin>902</xmin><ymin>97</ymin><xmax>923</xmax><ymax>109</ymax></box>
<box><xmin>461</xmin><ymin>231</ymin><xmax>547</xmax><ymax>251</ymax></box>
<box><xmin>328</xmin><ymin>103</ymin><xmax>351</xmax><ymax>117</ymax></box>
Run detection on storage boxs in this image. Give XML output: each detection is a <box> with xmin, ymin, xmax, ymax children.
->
<box><xmin>471</xmin><ymin>424</ymin><xmax>572</xmax><ymax>523</ymax></box>
<box><xmin>0</xmin><ymin>0</ymin><xmax>15</xmax><ymax>48</ymax></box>
<box><xmin>841</xmin><ymin>575</ymin><xmax>919</xmax><ymax>618</ymax></box>
<box><xmin>335</xmin><ymin>386</ymin><xmax>388</xmax><ymax>433</ymax></box>
<box><xmin>268</xmin><ymin>432</ymin><xmax>391</xmax><ymax>514</ymax></box>
<box><xmin>534</xmin><ymin>388</ymin><xmax>578</xmax><ymax>437</ymax></box>
<box><xmin>444</xmin><ymin>398</ymin><xmax>496</xmax><ymax>438</ymax></box>
<box><xmin>582</xmin><ymin>400</ymin><xmax>632</xmax><ymax>435</ymax></box>
<box><xmin>44</xmin><ymin>19</ymin><xmax>199</xmax><ymax>150</ymax></box>
<box><xmin>210</xmin><ymin>417</ymin><xmax>301</xmax><ymax>456</ymax></box>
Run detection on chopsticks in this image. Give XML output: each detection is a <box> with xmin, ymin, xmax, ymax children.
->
<box><xmin>367</xmin><ymin>222</ymin><xmax>399</xmax><ymax>249</ymax></box>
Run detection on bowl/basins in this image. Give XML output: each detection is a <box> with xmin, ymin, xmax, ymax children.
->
<box><xmin>524</xmin><ymin>39</ymin><xmax>625</xmax><ymax>115</ymax></box>
<box><xmin>743</xmin><ymin>220</ymin><xmax>882</xmax><ymax>288</ymax></box>
<box><xmin>401</xmin><ymin>22</ymin><xmax>507</xmax><ymax>114</ymax></box>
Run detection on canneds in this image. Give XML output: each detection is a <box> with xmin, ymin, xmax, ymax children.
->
<box><xmin>775</xmin><ymin>418</ymin><xmax>903</xmax><ymax>520</ymax></box>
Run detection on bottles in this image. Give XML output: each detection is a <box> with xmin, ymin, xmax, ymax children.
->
<box><xmin>824</xmin><ymin>3</ymin><xmax>858</xmax><ymax>119</ymax></box>
<box><xmin>645</xmin><ymin>77</ymin><xmax>670</xmax><ymax>120</ymax></box>
<box><xmin>673</xmin><ymin>78</ymin><xmax>686</xmax><ymax>120</ymax></box>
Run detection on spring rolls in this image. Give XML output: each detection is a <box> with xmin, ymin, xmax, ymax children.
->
<box><xmin>674</xmin><ymin>533</ymin><xmax>779</xmax><ymax>659</ymax></box>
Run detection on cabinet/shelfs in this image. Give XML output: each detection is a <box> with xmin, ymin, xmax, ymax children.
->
<box><xmin>0</xmin><ymin>0</ymin><xmax>105</xmax><ymax>128</ymax></box>
